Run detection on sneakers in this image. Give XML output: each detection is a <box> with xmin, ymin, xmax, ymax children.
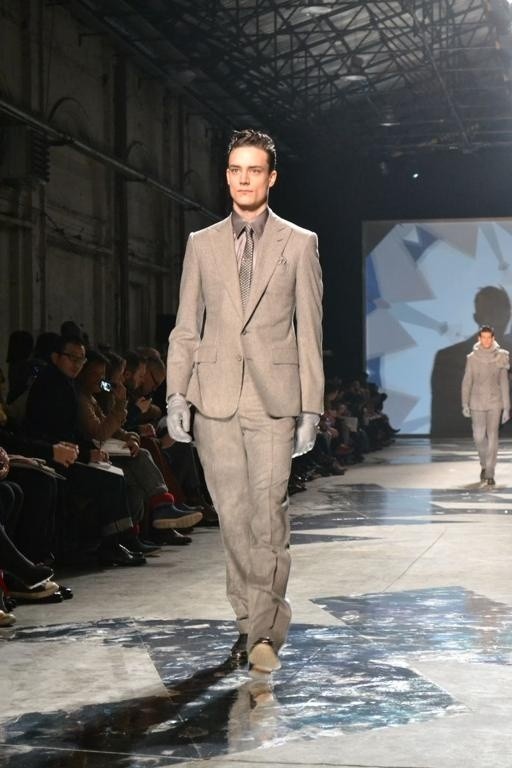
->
<box><xmin>480</xmin><ymin>468</ymin><xmax>487</xmax><ymax>481</ymax></box>
<box><xmin>112</xmin><ymin>537</ymin><xmax>161</xmax><ymax>557</ymax></box>
<box><xmin>0</xmin><ymin>609</ymin><xmax>16</xmax><ymax>626</ymax></box>
<box><xmin>151</xmin><ymin>510</ymin><xmax>203</xmax><ymax>529</ymax></box>
<box><xmin>487</xmin><ymin>477</ymin><xmax>495</xmax><ymax>486</ymax></box>
<box><xmin>173</xmin><ymin>502</ymin><xmax>204</xmax><ymax>512</ymax></box>
<box><xmin>192</xmin><ymin>502</ymin><xmax>218</xmax><ymax>521</ymax></box>
<box><xmin>0</xmin><ymin>578</ymin><xmax>59</xmax><ymax>599</ymax></box>
<box><xmin>292</xmin><ymin>428</ymin><xmax>402</xmax><ymax>493</ymax></box>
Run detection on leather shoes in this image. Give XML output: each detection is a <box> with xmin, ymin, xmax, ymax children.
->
<box><xmin>147</xmin><ymin>526</ymin><xmax>194</xmax><ymax>546</ymax></box>
<box><xmin>116</xmin><ymin>543</ymin><xmax>143</xmax><ymax>556</ymax></box>
<box><xmin>232</xmin><ymin>633</ymin><xmax>250</xmax><ymax>659</ymax></box>
<box><xmin>13</xmin><ymin>583</ymin><xmax>73</xmax><ymax>604</ymax></box>
<box><xmin>96</xmin><ymin>543</ymin><xmax>147</xmax><ymax>567</ymax></box>
<box><xmin>248</xmin><ymin>637</ymin><xmax>282</xmax><ymax>671</ymax></box>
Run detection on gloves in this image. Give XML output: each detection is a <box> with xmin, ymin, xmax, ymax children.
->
<box><xmin>165</xmin><ymin>394</ymin><xmax>194</xmax><ymax>444</ymax></box>
<box><xmin>501</xmin><ymin>408</ymin><xmax>511</xmax><ymax>425</ymax></box>
<box><xmin>461</xmin><ymin>407</ymin><xmax>472</xmax><ymax>418</ymax></box>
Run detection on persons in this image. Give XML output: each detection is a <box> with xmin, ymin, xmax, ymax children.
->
<box><xmin>458</xmin><ymin>324</ymin><xmax>511</xmax><ymax>487</ymax></box>
<box><xmin>160</xmin><ymin>123</ymin><xmax>327</xmax><ymax>675</ymax></box>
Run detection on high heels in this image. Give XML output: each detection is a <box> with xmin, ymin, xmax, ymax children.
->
<box><xmin>0</xmin><ymin>562</ymin><xmax>55</xmax><ymax>595</ymax></box>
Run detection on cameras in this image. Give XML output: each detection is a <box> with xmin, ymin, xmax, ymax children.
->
<box><xmin>99</xmin><ymin>379</ymin><xmax>116</xmax><ymax>393</ymax></box>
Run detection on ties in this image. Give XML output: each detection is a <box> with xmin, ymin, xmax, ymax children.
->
<box><xmin>237</xmin><ymin>223</ymin><xmax>257</xmax><ymax>314</ymax></box>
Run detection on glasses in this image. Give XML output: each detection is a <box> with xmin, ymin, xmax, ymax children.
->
<box><xmin>58</xmin><ymin>351</ymin><xmax>88</xmax><ymax>364</ymax></box>
